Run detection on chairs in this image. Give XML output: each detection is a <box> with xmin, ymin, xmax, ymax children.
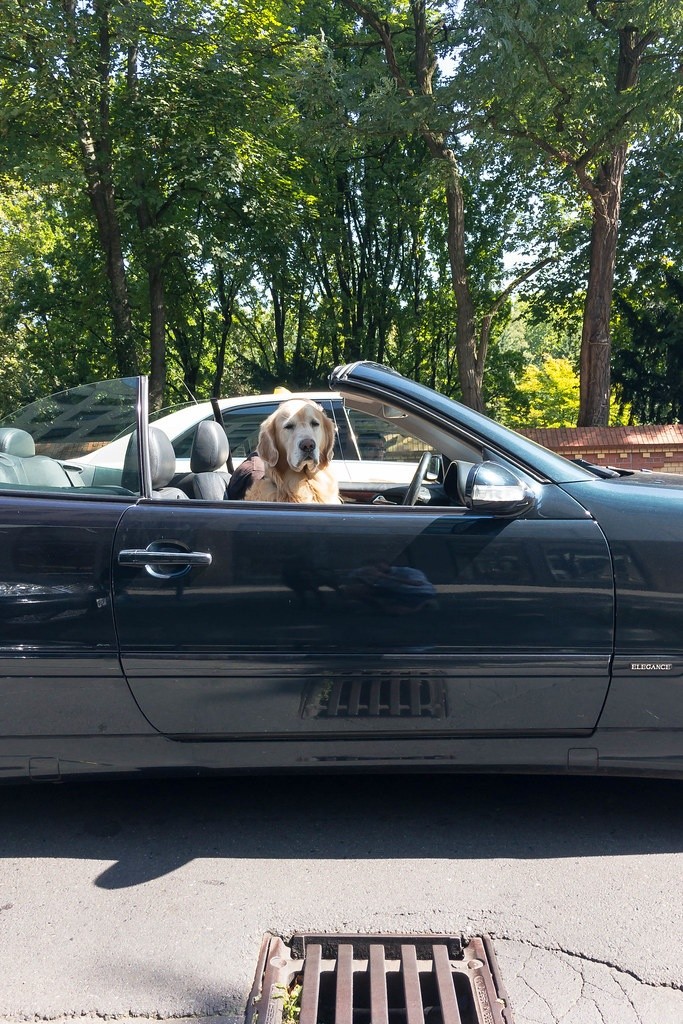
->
<box><xmin>122</xmin><ymin>421</ymin><xmax>191</xmax><ymax>501</ymax></box>
<box><xmin>0</xmin><ymin>425</ymin><xmax>74</xmax><ymax>489</ymax></box>
<box><xmin>175</xmin><ymin>420</ymin><xmax>240</xmax><ymax>503</ymax></box>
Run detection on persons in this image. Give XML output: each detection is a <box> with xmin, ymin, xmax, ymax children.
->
<box><xmin>355</xmin><ymin>432</ymin><xmax>387</xmax><ymax>461</ymax></box>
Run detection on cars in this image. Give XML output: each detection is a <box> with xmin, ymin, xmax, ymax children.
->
<box><xmin>0</xmin><ymin>361</ymin><xmax>679</xmax><ymax>787</ymax></box>
<box><xmin>60</xmin><ymin>385</ymin><xmax>436</xmax><ymax>489</ymax></box>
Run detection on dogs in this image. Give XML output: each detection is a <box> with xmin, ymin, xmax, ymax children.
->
<box><xmin>242</xmin><ymin>400</ymin><xmax>339</xmax><ymax>504</ymax></box>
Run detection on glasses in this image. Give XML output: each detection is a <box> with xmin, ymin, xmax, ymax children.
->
<box><xmin>363</xmin><ymin>447</ymin><xmax>387</xmax><ymax>453</ymax></box>
